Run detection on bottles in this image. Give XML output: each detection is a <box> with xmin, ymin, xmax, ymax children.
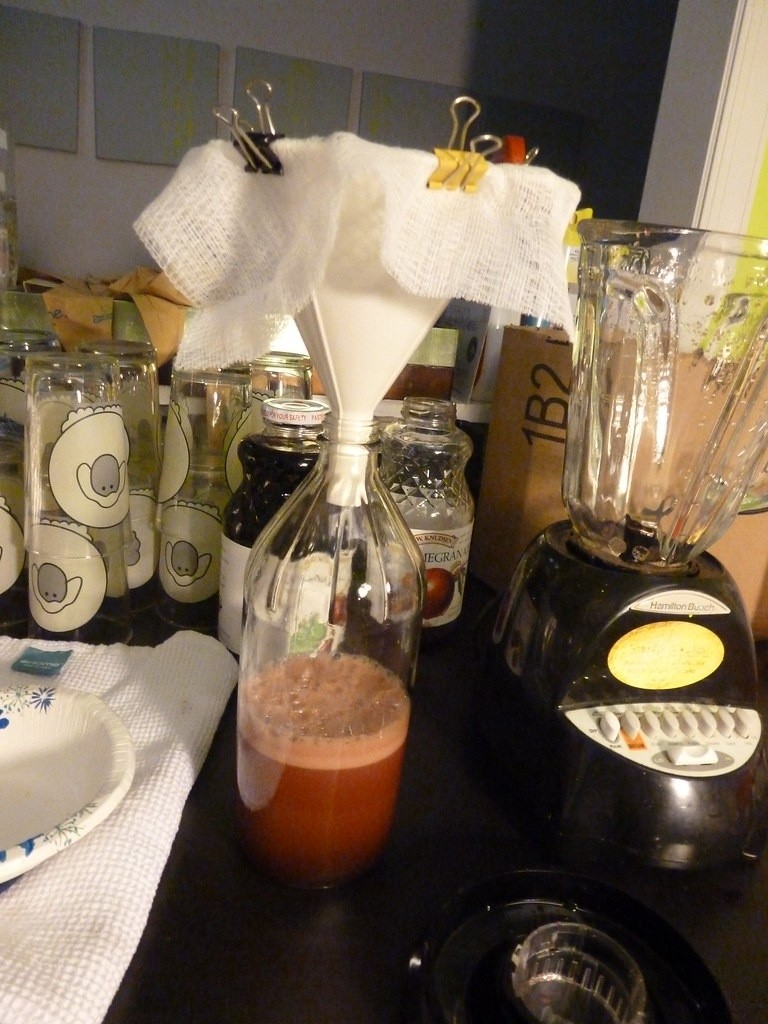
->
<box><xmin>382</xmin><ymin>396</ymin><xmax>475</xmax><ymax>634</ymax></box>
<box><xmin>234</xmin><ymin>416</ymin><xmax>425</xmax><ymax>886</ymax></box>
<box><xmin>219</xmin><ymin>394</ymin><xmax>353</xmax><ymax>655</ymax></box>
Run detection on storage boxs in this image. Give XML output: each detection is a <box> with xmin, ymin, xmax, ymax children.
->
<box><xmin>461</xmin><ymin>323</ymin><xmax>767</xmax><ymax>651</ymax></box>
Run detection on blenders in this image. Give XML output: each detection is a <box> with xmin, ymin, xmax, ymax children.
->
<box><xmin>486</xmin><ymin>220</ymin><xmax>767</xmax><ymax>913</ymax></box>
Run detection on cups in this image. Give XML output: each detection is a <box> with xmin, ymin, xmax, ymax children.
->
<box><xmin>0</xmin><ymin>127</ymin><xmax>313</xmax><ymax>645</ymax></box>
<box><xmin>512</xmin><ymin>924</ymin><xmax>647</xmax><ymax>1024</ymax></box>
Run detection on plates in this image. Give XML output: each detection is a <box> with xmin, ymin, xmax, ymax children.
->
<box><xmin>0</xmin><ymin>686</ymin><xmax>135</xmax><ymax>886</ymax></box>
<box><xmin>424</xmin><ymin>869</ymin><xmax>735</xmax><ymax>1024</ymax></box>
